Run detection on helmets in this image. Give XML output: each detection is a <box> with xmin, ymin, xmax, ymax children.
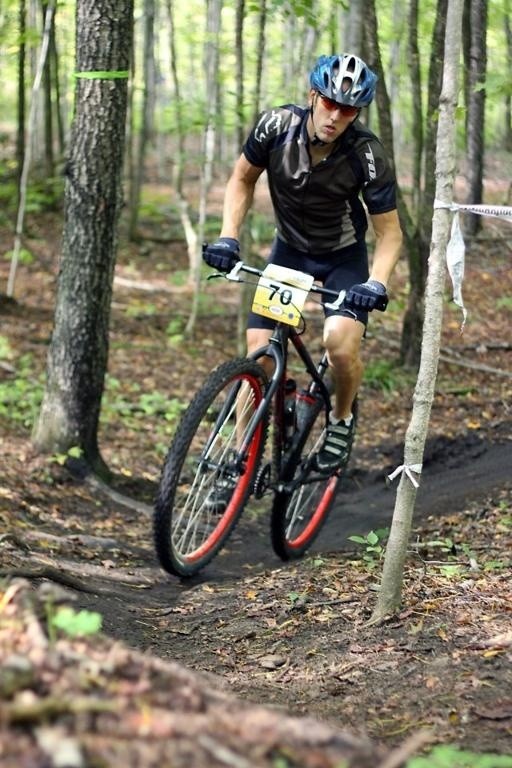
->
<box><xmin>309</xmin><ymin>53</ymin><xmax>378</xmax><ymax>107</ymax></box>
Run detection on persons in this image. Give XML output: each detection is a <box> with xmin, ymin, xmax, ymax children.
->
<box><xmin>204</xmin><ymin>47</ymin><xmax>405</xmax><ymax>513</ymax></box>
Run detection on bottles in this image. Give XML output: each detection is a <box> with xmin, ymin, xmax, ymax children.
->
<box><xmin>278</xmin><ymin>376</ymin><xmax>296</xmax><ymax>440</ymax></box>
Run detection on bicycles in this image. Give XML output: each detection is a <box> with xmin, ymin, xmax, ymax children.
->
<box><xmin>151</xmin><ymin>241</ymin><xmax>385</xmax><ymax>578</ymax></box>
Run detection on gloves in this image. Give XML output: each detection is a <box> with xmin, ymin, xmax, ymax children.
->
<box><xmin>203</xmin><ymin>237</ymin><xmax>241</xmax><ymax>273</ymax></box>
<box><xmin>344</xmin><ymin>279</ymin><xmax>389</xmax><ymax>313</ymax></box>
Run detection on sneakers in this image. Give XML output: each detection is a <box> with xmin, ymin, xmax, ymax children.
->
<box><xmin>314</xmin><ymin>411</ymin><xmax>354</xmax><ymax>473</ymax></box>
<box><xmin>202</xmin><ymin>446</ymin><xmax>248</xmax><ymax>513</ymax></box>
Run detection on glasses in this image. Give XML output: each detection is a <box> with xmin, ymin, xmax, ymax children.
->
<box><xmin>319</xmin><ymin>94</ymin><xmax>358</xmax><ymax>117</ymax></box>
